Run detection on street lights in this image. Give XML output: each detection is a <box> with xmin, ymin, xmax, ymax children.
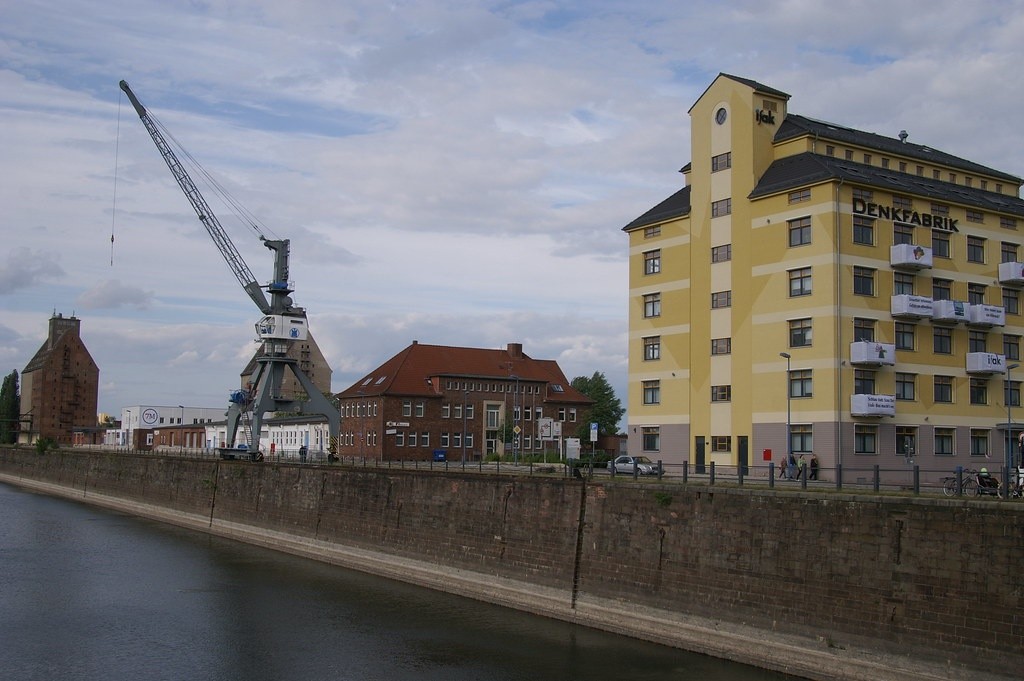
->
<box><xmin>462</xmin><ymin>391</ymin><xmax>470</xmax><ymax>470</ymax></box>
<box><xmin>779</xmin><ymin>352</ymin><xmax>791</xmax><ymax>480</ymax></box>
<box><xmin>511</xmin><ymin>375</ymin><xmax>519</xmax><ymax>467</ymax></box>
<box><xmin>178</xmin><ymin>405</ymin><xmax>184</xmax><ymax>455</ymax></box>
<box><xmin>1006</xmin><ymin>363</ymin><xmax>1020</xmax><ymax>500</ymax></box>
<box><xmin>126</xmin><ymin>410</ymin><xmax>131</xmax><ymax>452</ymax></box>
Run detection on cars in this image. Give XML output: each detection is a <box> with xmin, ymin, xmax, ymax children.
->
<box><xmin>606</xmin><ymin>455</ymin><xmax>666</xmax><ymax>477</ymax></box>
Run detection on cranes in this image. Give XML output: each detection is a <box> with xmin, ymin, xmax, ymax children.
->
<box><xmin>119</xmin><ymin>75</ymin><xmax>345</xmax><ymax>464</ymax></box>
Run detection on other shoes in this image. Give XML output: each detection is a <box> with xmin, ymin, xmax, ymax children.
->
<box><xmin>796</xmin><ymin>479</ymin><xmax>800</xmax><ymax>482</ymax></box>
<box><xmin>809</xmin><ymin>476</ymin><xmax>811</xmax><ymax>480</ymax></box>
<box><xmin>790</xmin><ymin>476</ymin><xmax>794</xmax><ymax>479</ymax></box>
<box><xmin>814</xmin><ymin>478</ymin><xmax>818</xmax><ymax>480</ymax></box>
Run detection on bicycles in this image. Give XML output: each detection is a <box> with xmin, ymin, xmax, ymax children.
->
<box><xmin>997</xmin><ymin>473</ymin><xmax>1024</xmax><ymax>501</ymax></box>
<box><xmin>942</xmin><ymin>468</ymin><xmax>980</xmax><ymax>498</ymax></box>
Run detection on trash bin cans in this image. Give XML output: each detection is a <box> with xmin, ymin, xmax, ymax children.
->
<box><xmin>433</xmin><ymin>450</ymin><xmax>447</xmax><ymax>461</ymax></box>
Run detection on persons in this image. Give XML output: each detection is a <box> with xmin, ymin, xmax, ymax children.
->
<box><xmin>778</xmin><ymin>457</ymin><xmax>788</xmax><ymax>479</ymax></box>
<box><xmin>790</xmin><ymin>452</ymin><xmax>796</xmax><ymax>478</ymax></box>
<box><xmin>797</xmin><ymin>454</ymin><xmax>806</xmax><ymax>480</ymax></box>
<box><xmin>809</xmin><ymin>455</ymin><xmax>818</xmax><ymax>480</ymax></box>
<box><xmin>299</xmin><ymin>445</ymin><xmax>306</xmax><ymax>463</ymax></box>
